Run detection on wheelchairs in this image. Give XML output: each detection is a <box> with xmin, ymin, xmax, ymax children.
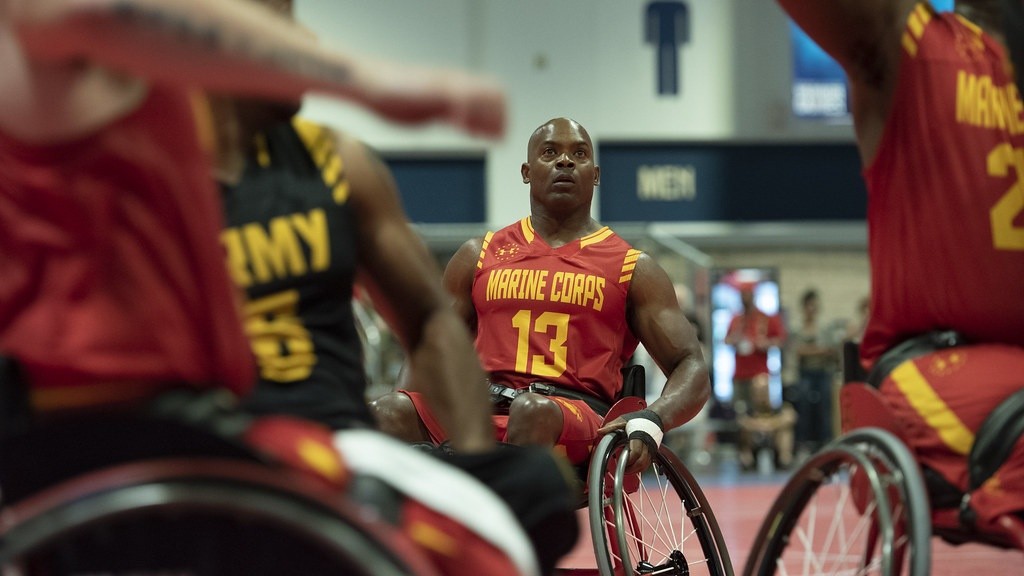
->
<box><xmin>744</xmin><ymin>341</ymin><xmax>937</xmax><ymax>576</ymax></box>
<box><xmin>1</xmin><ymin>364</ymin><xmax>738</xmax><ymax>576</ymax></box>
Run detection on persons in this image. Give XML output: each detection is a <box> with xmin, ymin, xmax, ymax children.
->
<box><xmin>784</xmin><ymin>291</ymin><xmax>838</xmax><ymax>451</ymax></box>
<box><xmin>725</xmin><ymin>284</ymin><xmax>787</xmax><ymax>470</ymax></box>
<box><xmin>0</xmin><ymin>0</ymin><xmax>583</xmax><ymax>576</ymax></box>
<box><xmin>368</xmin><ymin>116</ymin><xmax>712</xmax><ymax>475</ymax></box>
<box><xmin>775</xmin><ymin>0</ymin><xmax>1024</xmax><ymax>540</ymax></box>
